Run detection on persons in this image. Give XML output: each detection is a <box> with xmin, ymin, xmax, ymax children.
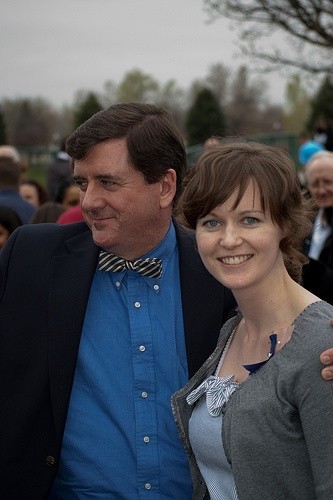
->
<box><xmin>0</xmin><ymin>136</ymin><xmax>85</xmax><ymax>250</ymax></box>
<box><xmin>0</xmin><ymin>104</ymin><xmax>333</xmax><ymax>499</ymax></box>
<box><xmin>298</xmin><ymin>117</ymin><xmax>333</xmax><ymax>169</ymax></box>
<box><xmin>171</xmin><ymin>141</ymin><xmax>333</xmax><ymax>499</ymax></box>
<box><xmin>297</xmin><ymin>151</ymin><xmax>333</xmax><ymax>306</ymax></box>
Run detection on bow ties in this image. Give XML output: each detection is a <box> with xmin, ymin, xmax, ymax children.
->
<box><xmin>97</xmin><ymin>250</ymin><xmax>163</xmax><ymax>280</ymax></box>
